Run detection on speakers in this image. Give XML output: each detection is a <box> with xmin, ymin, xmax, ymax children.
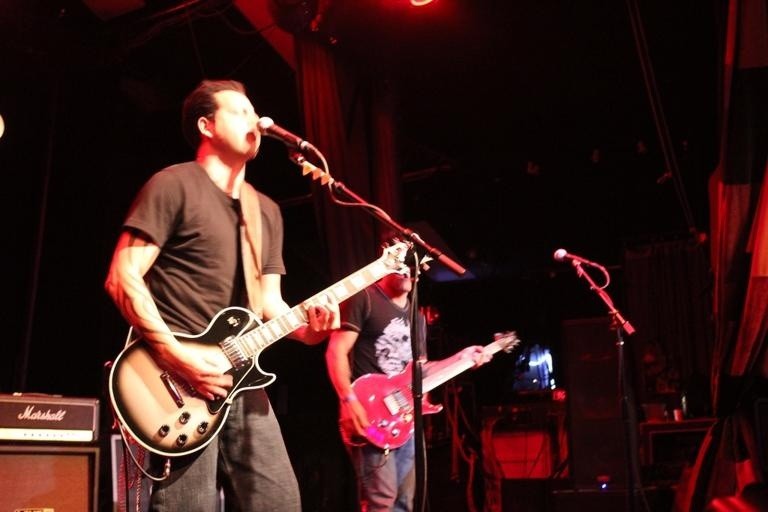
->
<box><xmin>550</xmin><ymin>489</ymin><xmax>639</xmax><ymax>512</ymax></box>
<box><xmin>556</xmin><ymin>315</ymin><xmax>642</xmax><ymax>418</ymax></box>
<box><xmin>0</xmin><ymin>391</ymin><xmax>154</xmax><ymax>512</ymax></box>
<box><xmin>567</xmin><ymin>419</ymin><xmax>633</xmax><ymax>490</ymax></box>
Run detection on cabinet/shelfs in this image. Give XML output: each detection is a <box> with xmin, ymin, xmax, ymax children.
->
<box><xmin>0</xmin><ymin>444</ymin><xmax>100</xmax><ymax>512</ymax></box>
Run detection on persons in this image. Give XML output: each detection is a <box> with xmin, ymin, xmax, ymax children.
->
<box><xmin>104</xmin><ymin>79</ymin><xmax>341</xmax><ymax>511</ymax></box>
<box><xmin>325</xmin><ymin>231</ymin><xmax>494</xmax><ymax>512</ymax></box>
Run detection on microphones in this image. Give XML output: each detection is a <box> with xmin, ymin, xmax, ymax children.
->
<box><xmin>553</xmin><ymin>248</ymin><xmax>599</xmax><ymax>270</ymax></box>
<box><xmin>256</xmin><ymin>116</ymin><xmax>309</xmax><ymax>152</ymax></box>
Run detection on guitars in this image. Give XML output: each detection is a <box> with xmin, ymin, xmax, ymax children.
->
<box><xmin>107</xmin><ymin>236</ymin><xmax>414</xmax><ymax>457</ymax></box>
<box><xmin>350</xmin><ymin>331</ymin><xmax>522</xmax><ymax>451</ymax></box>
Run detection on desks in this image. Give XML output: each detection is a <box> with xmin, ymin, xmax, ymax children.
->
<box><xmin>639</xmin><ymin>416</ymin><xmax>720</xmax><ymax>468</ymax></box>
